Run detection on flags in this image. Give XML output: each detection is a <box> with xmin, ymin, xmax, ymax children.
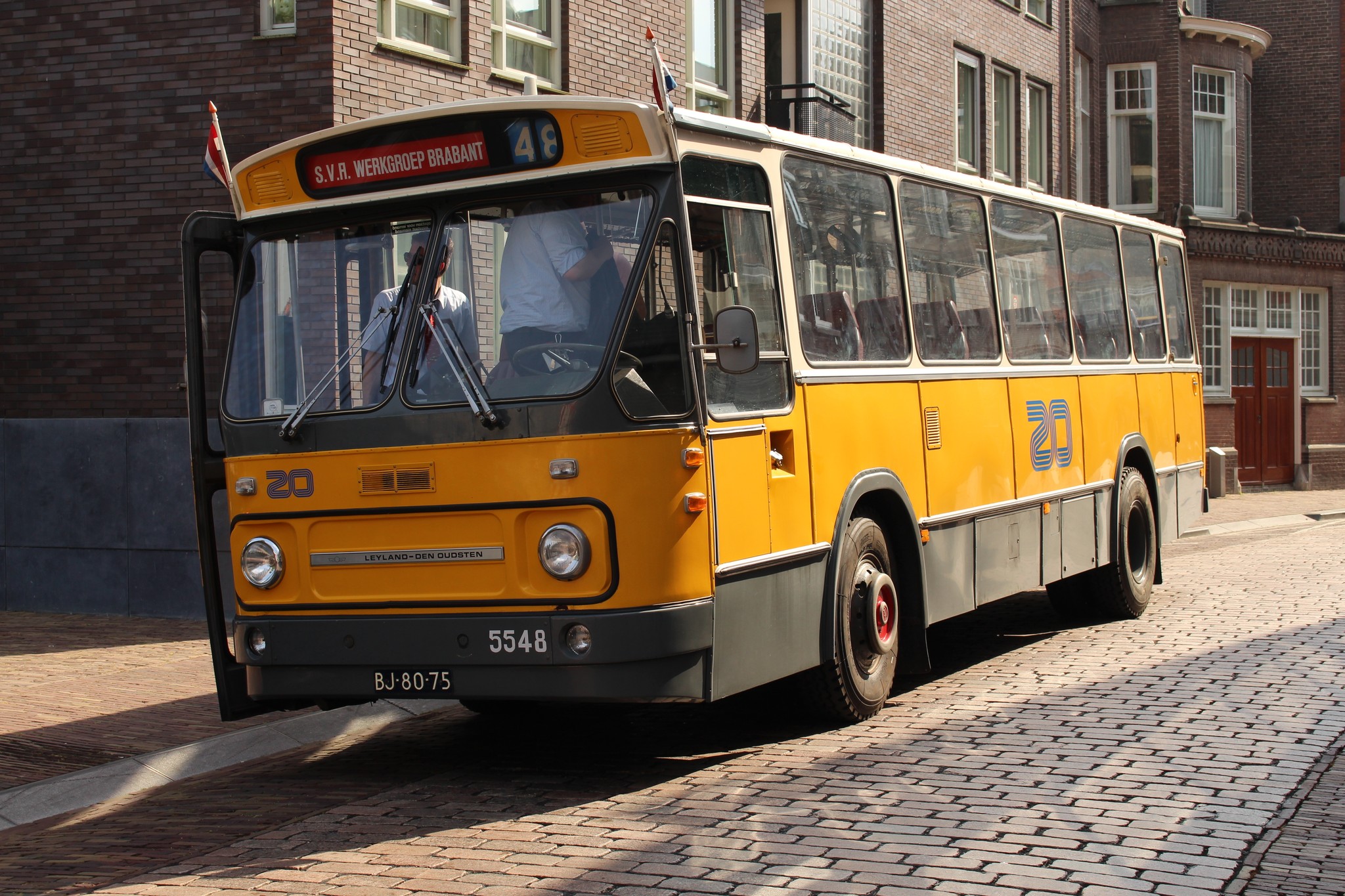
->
<box><xmin>651</xmin><ymin>50</ymin><xmax>679</xmax><ymax>114</ymax></box>
<box><xmin>201</xmin><ymin>121</ymin><xmax>231</xmax><ymax>192</ymax></box>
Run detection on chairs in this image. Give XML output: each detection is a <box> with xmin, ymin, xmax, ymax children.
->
<box><xmin>791</xmin><ymin>286</ymin><xmax>1173</xmax><ymax>364</ymax></box>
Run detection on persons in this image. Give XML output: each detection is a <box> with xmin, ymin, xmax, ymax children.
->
<box><xmin>359</xmin><ymin>231</ymin><xmax>479</xmax><ymax>405</ymax></box>
<box><xmin>498</xmin><ymin>191</ymin><xmax>648</xmax><ymax>376</ymax></box>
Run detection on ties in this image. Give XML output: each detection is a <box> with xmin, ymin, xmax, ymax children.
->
<box><xmin>424</xmin><ymin>301</ymin><xmax>441</xmax><ymax>370</ymax></box>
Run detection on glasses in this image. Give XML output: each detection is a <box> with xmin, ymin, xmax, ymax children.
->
<box><xmin>403</xmin><ymin>252</ymin><xmax>424</xmax><ymax>265</ymax></box>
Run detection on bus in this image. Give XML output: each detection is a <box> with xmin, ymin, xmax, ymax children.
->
<box><xmin>177</xmin><ymin>26</ymin><xmax>1211</xmax><ymax>730</ymax></box>
